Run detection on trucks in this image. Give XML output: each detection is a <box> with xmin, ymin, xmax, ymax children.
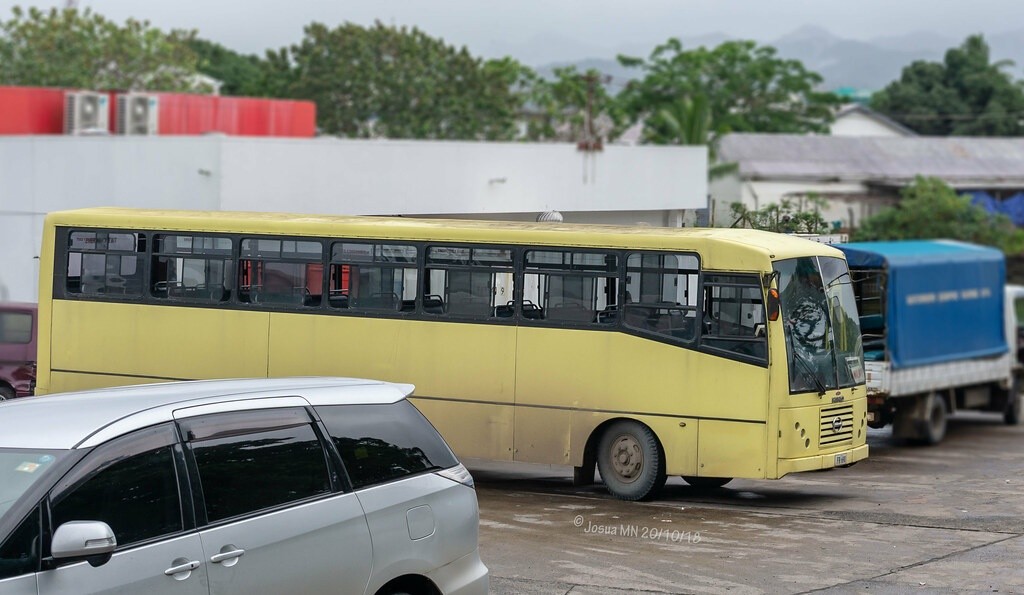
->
<box><xmin>823</xmin><ymin>239</ymin><xmax>1024</xmax><ymax>447</ymax></box>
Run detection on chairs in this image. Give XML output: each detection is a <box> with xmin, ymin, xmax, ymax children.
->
<box><xmin>77</xmin><ymin>274</ymin><xmax>711</xmax><ymax>344</ymax></box>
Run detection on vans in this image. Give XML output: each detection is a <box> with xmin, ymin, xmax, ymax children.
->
<box><xmin>0</xmin><ymin>301</ymin><xmax>38</xmax><ymax>401</ymax></box>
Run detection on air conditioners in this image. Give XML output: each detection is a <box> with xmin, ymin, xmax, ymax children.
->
<box><xmin>117</xmin><ymin>91</ymin><xmax>158</xmax><ymax>137</ymax></box>
<box><xmin>62</xmin><ymin>90</ymin><xmax>110</xmax><ymax>138</ymax></box>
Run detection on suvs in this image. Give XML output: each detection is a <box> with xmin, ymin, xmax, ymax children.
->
<box><xmin>0</xmin><ymin>376</ymin><xmax>489</xmax><ymax>595</ymax></box>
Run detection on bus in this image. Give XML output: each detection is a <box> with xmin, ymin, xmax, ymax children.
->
<box><xmin>34</xmin><ymin>206</ymin><xmax>869</xmax><ymax>501</ymax></box>
